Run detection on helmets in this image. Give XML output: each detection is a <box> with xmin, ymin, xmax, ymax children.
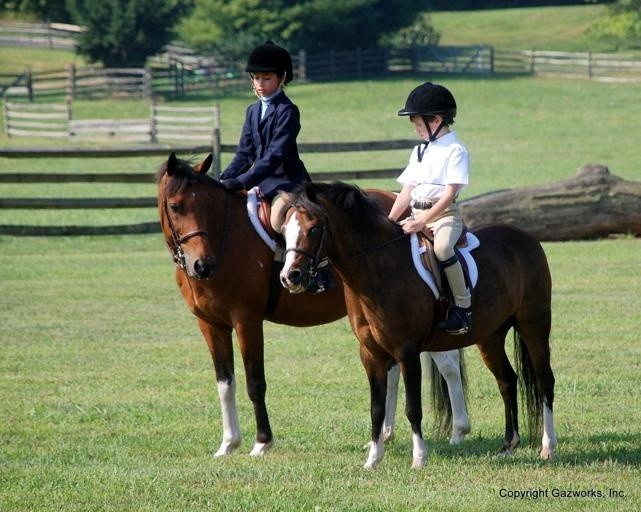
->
<box><xmin>396</xmin><ymin>81</ymin><xmax>457</xmax><ymax>117</ymax></box>
<box><xmin>243</xmin><ymin>41</ymin><xmax>293</xmax><ymax>83</ymax></box>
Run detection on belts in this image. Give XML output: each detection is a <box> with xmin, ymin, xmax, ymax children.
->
<box><xmin>410</xmin><ymin>197</ymin><xmax>456</xmax><ymax>211</ymax></box>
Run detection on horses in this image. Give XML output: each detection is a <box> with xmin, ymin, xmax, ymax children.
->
<box><xmin>152</xmin><ymin>149</ymin><xmax>473</xmax><ymax>459</ymax></box>
<box><xmin>275</xmin><ymin>176</ymin><xmax>558</xmax><ymax>473</ymax></box>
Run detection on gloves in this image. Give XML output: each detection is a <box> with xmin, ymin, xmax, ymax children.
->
<box><xmin>222</xmin><ymin>177</ymin><xmax>244</xmax><ymax>191</ymax></box>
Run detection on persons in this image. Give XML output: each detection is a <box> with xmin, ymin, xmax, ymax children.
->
<box><xmin>382</xmin><ymin>79</ymin><xmax>477</xmax><ymax>337</ymax></box>
<box><xmin>212</xmin><ymin>39</ymin><xmax>336</xmax><ymax>298</ymax></box>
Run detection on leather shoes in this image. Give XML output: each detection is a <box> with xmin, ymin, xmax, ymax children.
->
<box><xmin>445</xmin><ymin>303</ymin><xmax>477</xmax><ymax>338</ymax></box>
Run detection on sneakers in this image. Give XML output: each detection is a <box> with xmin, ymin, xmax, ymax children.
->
<box><xmin>309</xmin><ymin>267</ymin><xmax>337</xmax><ymax>295</ymax></box>
